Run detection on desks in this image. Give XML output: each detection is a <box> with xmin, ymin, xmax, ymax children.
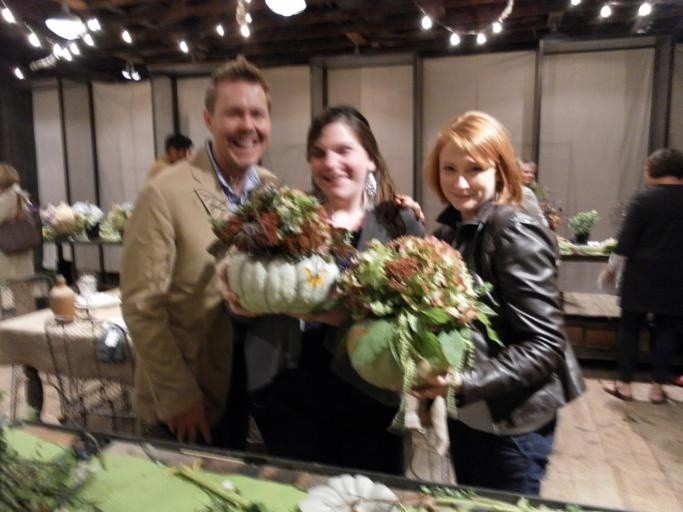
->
<box><xmin>1</xmin><ymin>290</ymin><xmax>133</xmax><ymax>423</ymax></box>
<box><xmin>561</xmin><ymin>289</ymin><xmax>622</xmax><ymax>372</ymax></box>
<box><xmin>58</xmin><ymin>236</ymin><xmax>122</xmax><ymax>288</ymax></box>
<box><xmin>2</xmin><ymin>420</ymin><xmax>620</xmax><ymax>512</ymax></box>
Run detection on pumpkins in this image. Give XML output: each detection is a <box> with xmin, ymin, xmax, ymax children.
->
<box><xmin>340</xmin><ymin>317</ymin><xmax>477</xmax><ymax>392</ymax></box>
<box><xmin>229</xmin><ymin>249</ymin><xmax>334</xmax><ymax>315</ymax></box>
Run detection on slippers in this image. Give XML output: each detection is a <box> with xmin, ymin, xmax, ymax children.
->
<box><xmin>603</xmin><ymin>379</ymin><xmax>636</xmax><ymax>401</ymax></box>
<box><xmin>649</xmin><ymin>382</ymin><xmax>668</xmax><ymax>406</ymax></box>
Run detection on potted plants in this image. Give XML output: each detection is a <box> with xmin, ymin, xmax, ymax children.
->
<box><xmin>567</xmin><ymin>209</ymin><xmax>599</xmax><ymax>244</ymax></box>
<box><xmin>210</xmin><ymin>185</ymin><xmax>340</xmax><ymax>317</ymax></box>
<box><xmin>73</xmin><ymin>200</ymin><xmax>103</xmax><ymax>240</ymax></box>
<box><xmin>345</xmin><ymin>235</ymin><xmax>505</xmax><ymax>393</ymax></box>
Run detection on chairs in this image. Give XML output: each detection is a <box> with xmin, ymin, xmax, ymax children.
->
<box><xmin>0</xmin><ymin>250</ymin><xmax>49</xmax><ymax>422</ymax></box>
<box><xmin>45</xmin><ymin>317</ymin><xmax>136</xmax><ymax>433</ymax></box>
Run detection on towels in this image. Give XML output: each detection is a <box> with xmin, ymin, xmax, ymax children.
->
<box><xmin>401</xmin><ymin>394</ymin><xmax>457</xmax><ymax>484</ymax></box>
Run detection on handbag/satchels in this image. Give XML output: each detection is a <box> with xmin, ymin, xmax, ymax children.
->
<box><xmin>1</xmin><ymin>216</ymin><xmax>44</xmax><ymax>255</ymax></box>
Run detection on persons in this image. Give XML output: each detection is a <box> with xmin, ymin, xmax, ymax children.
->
<box><xmin>119</xmin><ymin>54</ymin><xmax>426</xmax><ymax>448</ymax></box>
<box><xmin>0</xmin><ymin>161</ymin><xmax>37</xmax><ymax>316</ymax></box>
<box><xmin>148</xmin><ymin>133</ymin><xmax>193</xmax><ymax>180</ymax></box>
<box><xmin>407</xmin><ymin>110</ymin><xmax>587</xmax><ymax>497</ymax></box>
<box><xmin>519</xmin><ymin>159</ymin><xmax>537</xmax><ymax>191</ymax></box>
<box><xmin>216</xmin><ymin>104</ymin><xmax>429</xmax><ymax>477</ymax></box>
<box><xmin>597</xmin><ymin>149</ymin><xmax>683</xmax><ymax>403</ymax></box>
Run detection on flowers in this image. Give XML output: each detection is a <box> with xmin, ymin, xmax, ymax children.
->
<box><xmin>108</xmin><ymin>201</ymin><xmax>133</xmax><ymax>231</ymax></box>
<box><xmin>38</xmin><ymin>201</ymin><xmax>75</xmax><ymax>242</ymax></box>
